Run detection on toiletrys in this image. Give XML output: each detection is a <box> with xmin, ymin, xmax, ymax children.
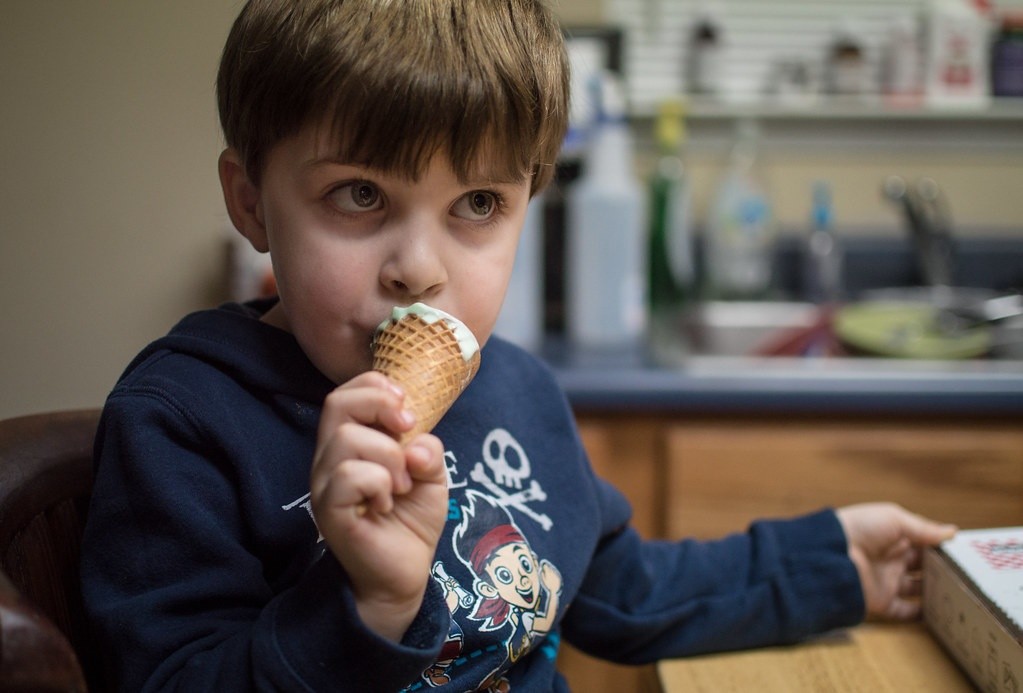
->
<box><xmin>646</xmin><ymin>98</ymin><xmax>794</xmax><ymax>302</ymax></box>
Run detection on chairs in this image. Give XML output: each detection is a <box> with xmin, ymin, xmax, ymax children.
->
<box><xmin>0</xmin><ymin>408</ymin><xmax>101</xmax><ymax>693</ymax></box>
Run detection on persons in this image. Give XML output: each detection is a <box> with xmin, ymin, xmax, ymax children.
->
<box><xmin>73</xmin><ymin>0</ymin><xmax>959</xmax><ymax>693</ymax></box>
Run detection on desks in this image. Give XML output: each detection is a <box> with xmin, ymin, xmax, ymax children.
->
<box><xmin>653</xmin><ymin>596</ymin><xmax>979</xmax><ymax>693</ymax></box>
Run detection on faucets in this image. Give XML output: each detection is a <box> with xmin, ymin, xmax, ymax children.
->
<box><xmin>875</xmin><ymin>181</ymin><xmax>960</xmax><ymax>283</ymax></box>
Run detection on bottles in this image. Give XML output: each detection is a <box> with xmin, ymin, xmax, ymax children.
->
<box><xmin>708</xmin><ymin>123</ymin><xmax>771</xmax><ymax>294</ymax></box>
<box><xmin>562</xmin><ymin>71</ymin><xmax>651</xmax><ymax>355</ymax></box>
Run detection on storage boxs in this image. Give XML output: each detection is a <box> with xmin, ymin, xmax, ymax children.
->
<box><xmin>919</xmin><ymin>526</ymin><xmax>1023</xmax><ymax>693</ymax></box>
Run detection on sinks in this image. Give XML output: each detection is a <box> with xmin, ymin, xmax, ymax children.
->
<box><xmin>660</xmin><ymin>304</ymin><xmax>1023</xmax><ymax>383</ymax></box>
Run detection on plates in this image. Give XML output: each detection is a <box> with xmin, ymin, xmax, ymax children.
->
<box><xmin>832</xmin><ymin>299</ymin><xmax>995</xmax><ymax>359</ymax></box>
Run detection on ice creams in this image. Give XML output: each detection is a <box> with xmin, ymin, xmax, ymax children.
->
<box><xmin>356</xmin><ymin>301</ymin><xmax>480</xmax><ymax>518</ymax></box>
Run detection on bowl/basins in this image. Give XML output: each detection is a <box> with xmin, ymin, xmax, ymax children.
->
<box><xmin>686</xmin><ymin>302</ymin><xmax>820</xmax><ymax>358</ymax></box>
